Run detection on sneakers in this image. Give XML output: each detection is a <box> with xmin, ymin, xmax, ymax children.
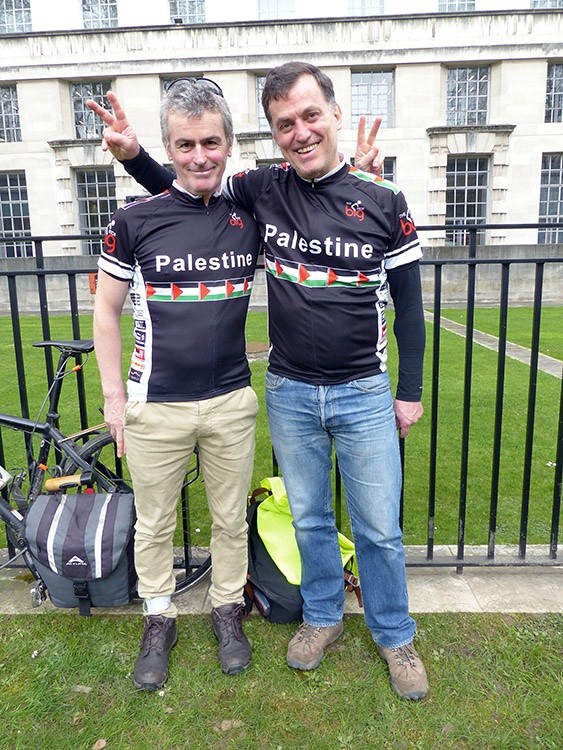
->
<box><xmin>375</xmin><ymin>640</ymin><xmax>430</xmax><ymax>699</ymax></box>
<box><xmin>286</xmin><ymin>619</ymin><xmax>343</xmax><ymax>670</ymax></box>
<box><xmin>133</xmin><ymin>613</ymin><xmax>178</xmax><ymax>691</ymax></box>
<box><xmin>210</xmin><ymin>603</ymin><xmax>252</xmax><ymax>674</ymax></box>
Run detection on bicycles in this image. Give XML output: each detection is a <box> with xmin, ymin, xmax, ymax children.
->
<box><xmin>1</xmin><ymin>337</ymin><xmax>218</xmax><ymax>599</ymax></box>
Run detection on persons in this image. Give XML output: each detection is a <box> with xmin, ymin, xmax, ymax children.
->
<box><xmin>92</xmin><ymin>77</ymin><xmax>384</xmax><ymax>688</ymax></box>
<box><xmin>85</xmin><ymin>60</ymin><xmax>429</xmax><ymax>702</ymax></box>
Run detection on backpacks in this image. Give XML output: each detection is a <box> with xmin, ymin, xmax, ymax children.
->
<box><xmin>24</xmin><ymin>493</ymin><xmax>134</xmax><ymax>609</ymax></box>
<box><xmin>247</xmin><ymin>486</ymin><xmax>363</xmax><ymax>624</ymax></box>
